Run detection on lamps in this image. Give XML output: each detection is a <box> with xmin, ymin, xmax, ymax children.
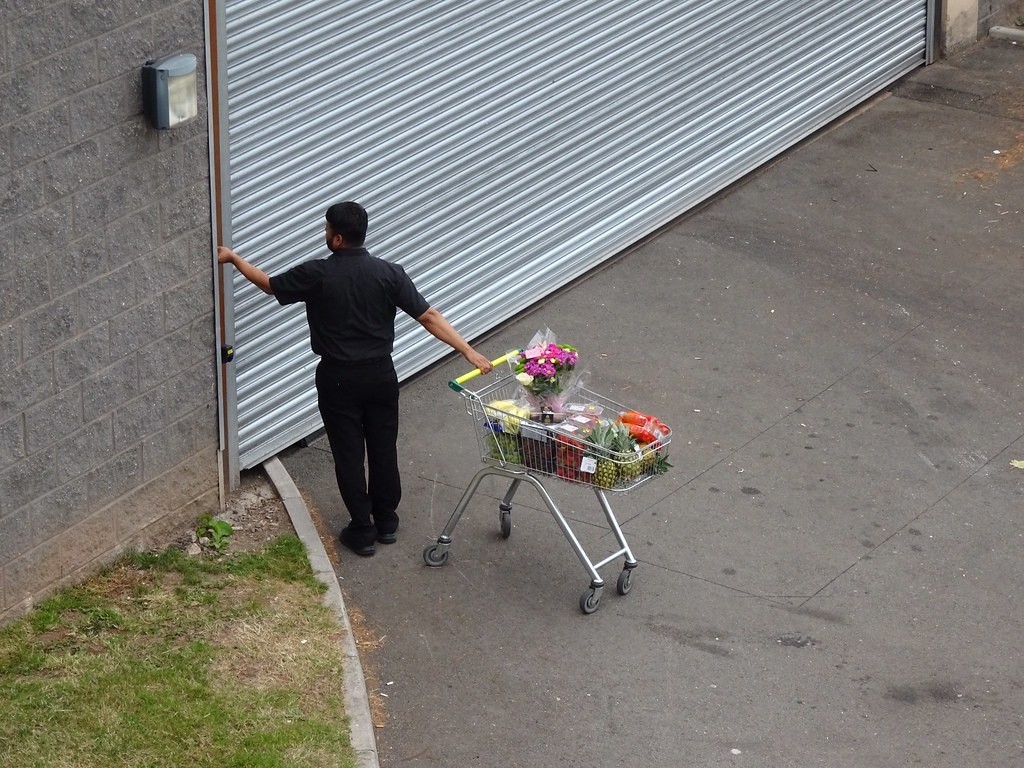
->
<box><xmin>142</xmin><ymin>53</ymin><xmax>198</xmax><ymax>130</ymax></box>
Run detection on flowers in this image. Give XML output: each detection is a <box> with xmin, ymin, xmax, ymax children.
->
<box><xmin>513</xmin><ymin>342</ymin><xmax>579</xmax><ymax>402</ymax></box>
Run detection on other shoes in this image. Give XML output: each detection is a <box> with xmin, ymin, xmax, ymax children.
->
<box><xmin>374</xmin><ymin>524</ymin><xmax>396</xmax><ymax>544</ymax></box>
<box><xmin>341</xmin><ymin>526</ymin><xmax>375</xmax><ymax>555</ymax></box>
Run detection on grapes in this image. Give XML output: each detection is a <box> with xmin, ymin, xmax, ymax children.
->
<box><xmin>485</xmin><ymin>432</ymin><xmax>522</xmax><ymax>464</ymax></box>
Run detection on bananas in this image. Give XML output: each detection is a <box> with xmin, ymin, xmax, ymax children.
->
<box><xmin>484</xmin><ymin>400</ymin><xmax>530</xmax><ymax>434</ymax></box>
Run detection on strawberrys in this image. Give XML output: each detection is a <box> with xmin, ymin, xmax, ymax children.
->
<box><xmin>556</xmin><ymin>415</ymin><xmax>599</xmax><ymax>483</ymax></box>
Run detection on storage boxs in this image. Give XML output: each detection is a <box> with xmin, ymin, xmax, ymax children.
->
<box><xmin>483</xmin><ymin>403</ymin><xmax>604</xmax><ymax>480</ymax></box>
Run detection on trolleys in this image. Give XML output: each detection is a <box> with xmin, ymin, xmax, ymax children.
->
<box><xmin>423</xmin><ymin>348</ymin><xmax>672</xmax><ymax>613</ymax></box>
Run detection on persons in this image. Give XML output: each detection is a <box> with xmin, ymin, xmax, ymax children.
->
<box><xmin>217</xmin><ymin>200</ymin><xmax>493</xmax><ymax>557</ymax></box>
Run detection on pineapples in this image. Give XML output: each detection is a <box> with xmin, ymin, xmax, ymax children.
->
<box><xmin>584</xmin><ymin>419</ymin><xmax>674</xmax><ymax>489</ymax></box>
